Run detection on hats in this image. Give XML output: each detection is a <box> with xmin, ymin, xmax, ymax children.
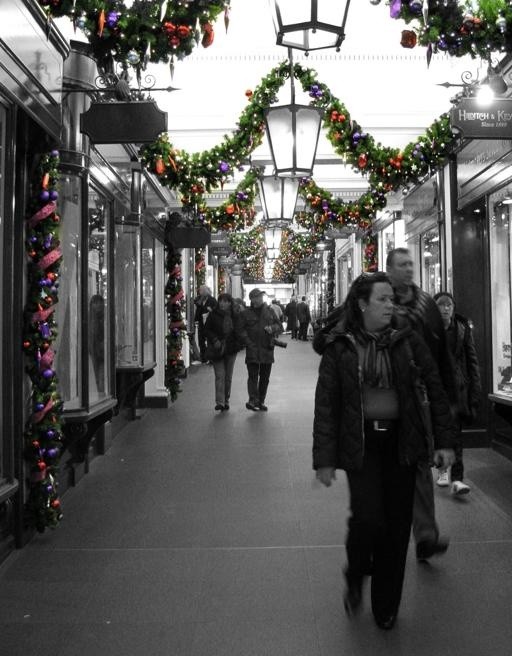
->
<box><xmin>432</xmin><ymin>292</ymin><xmax>456</xmax><ymax>310</ymax></box>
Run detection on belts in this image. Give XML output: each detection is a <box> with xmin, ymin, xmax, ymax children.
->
<box><xmin>364</xmin><ymin>419</ymin><xmax>402</xmax><ymax>433</ymax></box>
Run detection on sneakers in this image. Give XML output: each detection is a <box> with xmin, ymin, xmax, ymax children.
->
<box><xmin>343</xmin><ymin>584</ymin><xmax>398</xmax><ymax>630</ymax></box>
<box><xmin>215</xmin><ymin>404</ymin><xmax>229</xmax><ymax>412</ymax></box>
<box><xmin>435</xmin><ymin>467</ymin><xmax>471</xmax><ymax>497</ymax></box>
<box><xmin>415</xmin><ymin>538</ymin><xmax>448</xmax><ymax>561</ymax></box>
<box><xmin>245</xmin><ymin>401</ymin><xmax>267</xmax><ymax>411</ymax></box>
<box><xmin>249</xmin><ymin>288</ymin><xmax>265</xmax><ymax>300</ymax></box>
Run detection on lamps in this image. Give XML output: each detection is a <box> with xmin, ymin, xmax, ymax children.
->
<box><xmin>254</xmin><ymin>0</ymin><xmax>354</xmax><ymax>286</ymax></box>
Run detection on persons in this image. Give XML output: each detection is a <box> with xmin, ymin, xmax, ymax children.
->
<box><xmin>432</xmin><ymin>292</ymin><xmax>483</xmax><ymax>498</ymax></box>
<box><xmin>195</xmin><ymin>280</ymin><xmax>312</xmax><ymax>413</ymax></box>
<box><xmin>385</xmin><ymin>248</ymin><xmax>463</xmax><ymax>562</ymax></box>
<box><xmin>313</xmin><ymin>270</ymin><xmax>456</xmax><ymax>631</ymax></box>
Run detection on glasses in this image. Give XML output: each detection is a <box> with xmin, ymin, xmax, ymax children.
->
<box><xmin>357</xmin><ymin>270</ymin><xmax>389</xmax><ymax>288</ymax></box>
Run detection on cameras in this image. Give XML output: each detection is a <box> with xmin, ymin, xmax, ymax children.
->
<box><xmin>268</xmin><ymin>338</ymin><xmax>287</xmax><ymax>349</ymax></box>
